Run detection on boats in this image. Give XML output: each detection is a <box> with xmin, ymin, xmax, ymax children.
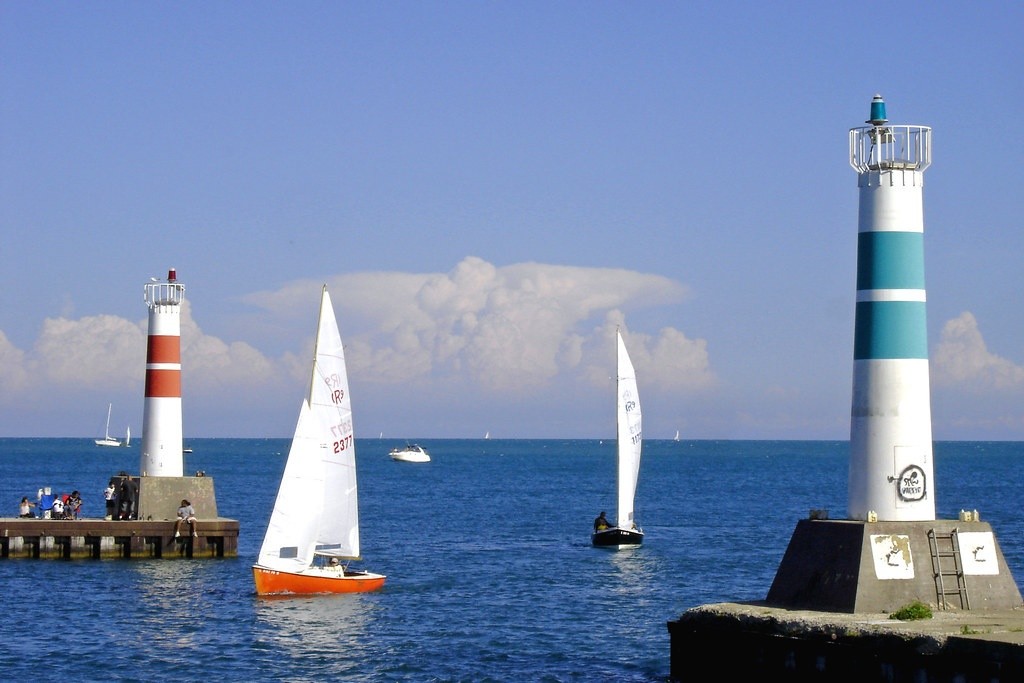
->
<box><xmin>388</xmin><ymin>443</ymin><xmax>431</xmax><ymax>463</ymax></box>
<box><xmin>183</xmin><ymin>448</ymin><xmax>192</xmax><ymax>453</ymax></box>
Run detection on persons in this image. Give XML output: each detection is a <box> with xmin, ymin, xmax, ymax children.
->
<box><xmin>393</xmin><ymin>447</ymin><xmax>398</xmax><ymax>453</ymax></box>
<box><xmin>594</xmin><ymin>511</ymin><xmax>615</xmax><ymax>532</ymax></box>
<box><xmin>65</xmin><ymin>490</ymin><xmax>82</xmax><ymax>519</ymax></box>
<box><xmin>18</xmin><ymin>499</ymin><xmax>37</xmax><ymax>518</ymax></box>
<box><xmin>53</xmin><ymin>494</ymin><xmax>65</xmax><ymax>520</ymax></box>
<box><xmin>175</xmin><ymin>498</ymin><xmax>201</xmax><ymax>539</ymax></box>
<box><xmin>119</xmin><ymin>474</ymin><xmax>138</xmax><ymax>520</ymax></box>
<box><xmin>103</xmin><ymin>481</ymin><xmax>118</xmax><ymax>521</ymax></box>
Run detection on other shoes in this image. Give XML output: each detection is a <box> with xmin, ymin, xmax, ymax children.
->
<box><xmin>174</xmin><ymin>532</ymin><xmax>198</xmax><ymax>538</ymax></box>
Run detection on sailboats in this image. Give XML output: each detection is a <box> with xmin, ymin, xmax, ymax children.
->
<box><xmin>588</xmin><ymin>323</ymin><xmax>646</xmax><ymax>552</ymax></box>
<box><xmin>249</xmin><ymin>281</ymin><xmax>387</xmax><ymax>598</ymax></box>
<box><xmin>94</xmin><ymin>402</ymin><xmax>131</xmax><ymax>447</ymax></box>
